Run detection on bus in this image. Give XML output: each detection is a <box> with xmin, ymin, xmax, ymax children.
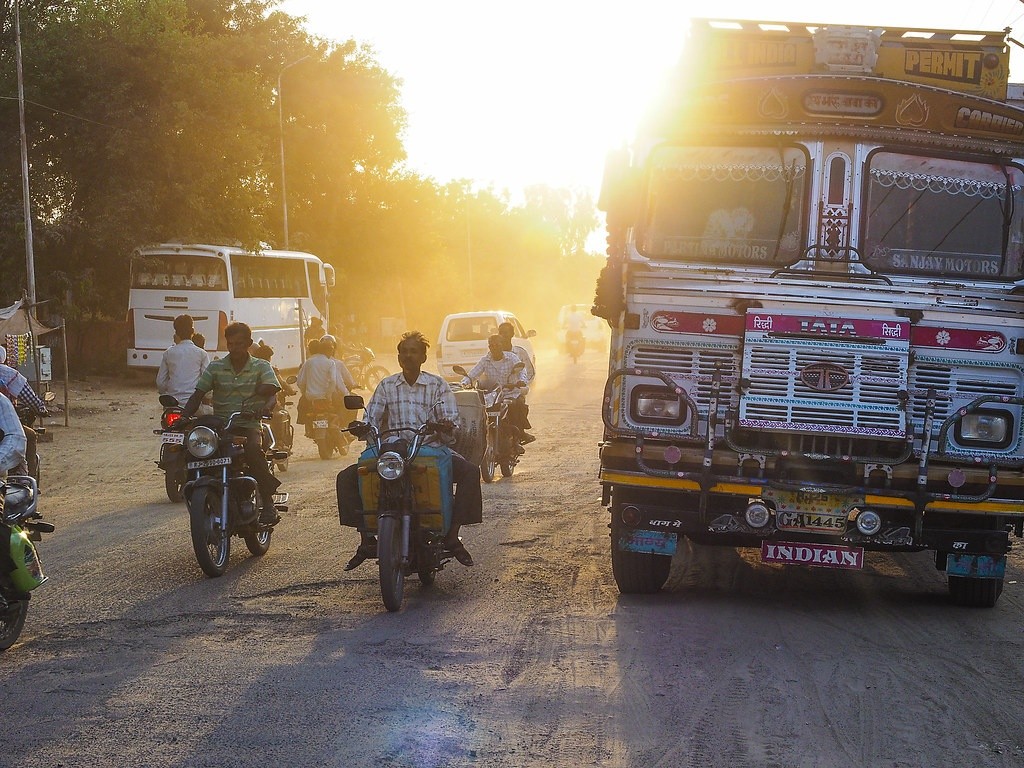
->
<box><xmin>126</xmin><ymin>243</ymin><xmax>335</xmax><ymax>371</ymax></box>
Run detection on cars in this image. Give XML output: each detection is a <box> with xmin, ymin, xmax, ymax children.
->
<box><xmin>558</xmin><ymin>304</ymin><xmax>608</xmax><ymax>354</ymax></box>
<box><xmin>436</xmin><ymin>311</ymin><xmax>537</xmax><ymax>381</ymax></box>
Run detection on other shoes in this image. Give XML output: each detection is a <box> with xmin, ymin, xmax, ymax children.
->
<box><xmin>343</xmin><ymin>538</ymin><xmax>380</xmax><ymax>571</ymax></box>
<box><xmin>517</xmin><ymin>432</ymin><xmax>536</xmax><ymax>442</ymax></box>
<box><xmin>513</xmin><ymin>444</ymin><xmax>525</xmax><ymax>454</ymax></box>
<box><xmin>444</xmin><ymin>536</ymin><xmax>475</xmax><ymax>567</ymax></box>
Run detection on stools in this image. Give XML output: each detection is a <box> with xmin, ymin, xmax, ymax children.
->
<box><xmin>29</xmin><ymin>382</ymin><xmax>49</xmax><ymax>404</ymax></box>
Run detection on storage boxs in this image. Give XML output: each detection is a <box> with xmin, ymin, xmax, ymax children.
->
<box><xmin>440</xmin><ymin>389</ymin><xmax>488</xmax><ymax>466</ymax></box>
<box><xmin>358</xmin><ymin>441</ymin><xmax>454</xmax><ymax>536</ymax></box>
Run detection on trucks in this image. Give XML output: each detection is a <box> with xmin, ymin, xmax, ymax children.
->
<box><xmin>597</xmin><ymin>20</ymin><xmax>1024</xmax><ymax>593</ymax></box>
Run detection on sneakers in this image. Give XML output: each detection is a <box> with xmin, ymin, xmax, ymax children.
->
<box><xmin>258</xmin><ymin>503</ymin><xmax>278</xmax><ymax>523</ymax></box>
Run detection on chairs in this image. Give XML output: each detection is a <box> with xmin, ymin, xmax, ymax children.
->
<box><xmin>139</xmin><ymin>272</ymin><xmax>223</xmax><ymax>288</ymax></box>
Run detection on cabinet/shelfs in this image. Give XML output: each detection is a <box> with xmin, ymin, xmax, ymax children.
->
<box><xmin>17</xmin><ymin>347</ymin><xmax>51</xmax><ymax>381</ymax></box>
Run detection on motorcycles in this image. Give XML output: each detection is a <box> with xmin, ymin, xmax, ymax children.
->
<box><xmin>452</xmin><ymin>361</ymin><xmax>535</xmax><ymax>482</ymax></box>
<box><xmin>563</xmin><ymin>325</ymin><xmax>587</xmax><ymax>364</ymax></box>
<box><xmin>153</xmin><ymin>356</ymin><xmax>219</xmax><ymax>501</ymax></box>
<box><xmin>299</xmin><ymin>385</ymin><xmax>364</xmax><ymax>460</ymax></box>
<box><xmin>0</xmin><ymin>475</ymin><xmax>55</xmax><ymax>651</ymax></box>
<box><xmin>158</xmin><ymin>382</ymin><xmax>289</xmax><ymax>576</ymax></box>
<box><xmin>335</xmin><ymin>394</ymin><xmax>463</xmax><ymax>610</ymax></box>
<box><xmin>337</xmin><ymin>341</ymin><xmax>390</xmax><ymax>392</ymax></box>
<box><xmin>263</xmin><ymin>376</ymin><xmax>298</xmax><ymax>472</ymax></box>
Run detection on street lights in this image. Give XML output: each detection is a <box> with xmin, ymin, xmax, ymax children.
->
<box><xmin>277</xmin><ymin>54</ymin><xmax>311</xmax><ymax>250</ymax></box>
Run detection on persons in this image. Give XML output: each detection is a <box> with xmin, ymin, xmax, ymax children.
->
<box><xmin>497</xmin><ymin>322</ymin><xmax>535</xmax><ymax>444</ymax></box>
<box><xmin>336</xmin><ymin>330</ymin><xmax>483</xmax><ymax>572</ymax></box>
<box><xmin>564</xmin><ymin>305</ymin><xmax>586</xmax><ymax>357</ymax></box>
<box><xmin>171</xmin><ymin>321</ymin><xmax>281</xmax><ymax>523</ymax></box>
<box><xmin>298</xmin><ymin>333</ymin><xmax>358</xmax><ymax>443</ymax></box>
<box><xmin>253</xmin><ymin>344</ymin><xmax>297</xmax><ymax>453</ymax></box>
<box><xmin>0</xmin><ymin>393</ymin><xmax>27</xmax><ymax>613</ymax></box>
<box><xmin>0</xmin><ymin>345</ymin><xmax>49</xmax><ymax>494</ymax></box>
<box><xmin>155</xmin><ymin>313</ymin><xmax>213</xmax><ymax>415</ymax></box>
<box><xmin>460</xmin><ymin>334</ymin><xmax>528</xmax><ymax>454</ymax></box>
